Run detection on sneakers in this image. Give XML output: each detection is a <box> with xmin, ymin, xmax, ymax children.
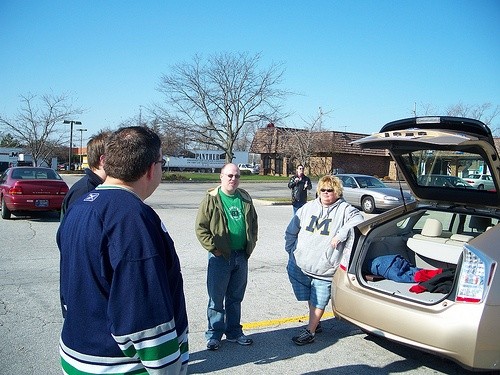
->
<box><xmin>206</xmin><ymin>338</ymin><xmax>222</xmax><ymax>349</ymax></box>
<box><xmin>294</xmin><ymin>329</ymin><xmax>315</xmax><ymax>345</ymax></box>
<box><xmin>305</xmin><ymin>323</ymin><xmax>322</xmax><ymax>333</ymax></box>
<box><xmin>227</xmin><ymin>333</ymin><xmax>252</xmax><ymax>345</ymax></box>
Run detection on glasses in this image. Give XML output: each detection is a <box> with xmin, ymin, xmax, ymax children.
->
<box><xmin>149</xmin><ymin>159</ymin><xmax>166</xmax><ymax>167</ymax></box>
<box><xmin>221</xmin><ymin>173</ymin><xmax>240</xmax><ymax>179</ymax></box>
<box><xmin>298</xmin><ymin>168</ymin><xmax>304</xmax><ymax>170</ymax></box>
<box><xmin>320</xmin><ymin>189</ymin><xmax>334</xmax><ymax>192</ymax></box>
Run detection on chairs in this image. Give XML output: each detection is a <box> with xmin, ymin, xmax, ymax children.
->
<box><xmin>469</xmin><ymin>216</ymin><xmax>492</xmax><ymax>233</ymax></box>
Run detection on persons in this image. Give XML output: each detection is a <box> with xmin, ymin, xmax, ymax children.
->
<box><xmin>57</xmin><ymin>126</ymin><xmax>189</xmax><ymax>375</ymax></box>
<box><xmin>195</xmin><ymin>163</ymin><xmax>258</xmax><ymax>351</ymax></box>
<box><xmin>288</xmin><ymin>165</ymin><xmax>312</xmax><ymax>214</ymax></box>
<box><xmin>285</xmin><ymin>175</ymin><xmax>365</xmax><ymax>346</ymax></box>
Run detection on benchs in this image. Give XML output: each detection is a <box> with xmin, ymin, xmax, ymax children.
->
<box><xmin>405</xmin><ymin>219</ymin><xmax>492</xmax><ymax>270</ymax></box>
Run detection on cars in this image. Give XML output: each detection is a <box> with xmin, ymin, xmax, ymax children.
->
<box><xmin>455</xmin><ymin>173</ymin><xmax>496</xmax><ymax>191</ymax></box>
<box><xmin>238</xmin><ymin>163</ymin><xmax>259</xmax><ymax>175</ymax></box>
<box><xmin>417</xmin><ymin>174</ymin><xmax>475</xmax><ymax>189</ymax></box>
<box><xmin>0</xmin><ymin>166</ymin><xmax>69</xmax><ymax>220</ymax></box>
<box><xmin>329</xmin><ymin>114</ymin><xmax>500</xmax><ymax>375</ymax></box>
<box><xmin>335</xmin><ymin>172</ymin><xmax>416</xmax><ymax>215</ymax></box>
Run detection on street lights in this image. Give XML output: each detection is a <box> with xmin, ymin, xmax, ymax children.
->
<box><xmin>76</xmin><ymin>129</ymin><xmax>87</xmax><ymax>171</ymax></box>
<box><xmin>64</xmin><ymin>119</ymin><xmax>82</xmax><ymax>174</ymax></box>
